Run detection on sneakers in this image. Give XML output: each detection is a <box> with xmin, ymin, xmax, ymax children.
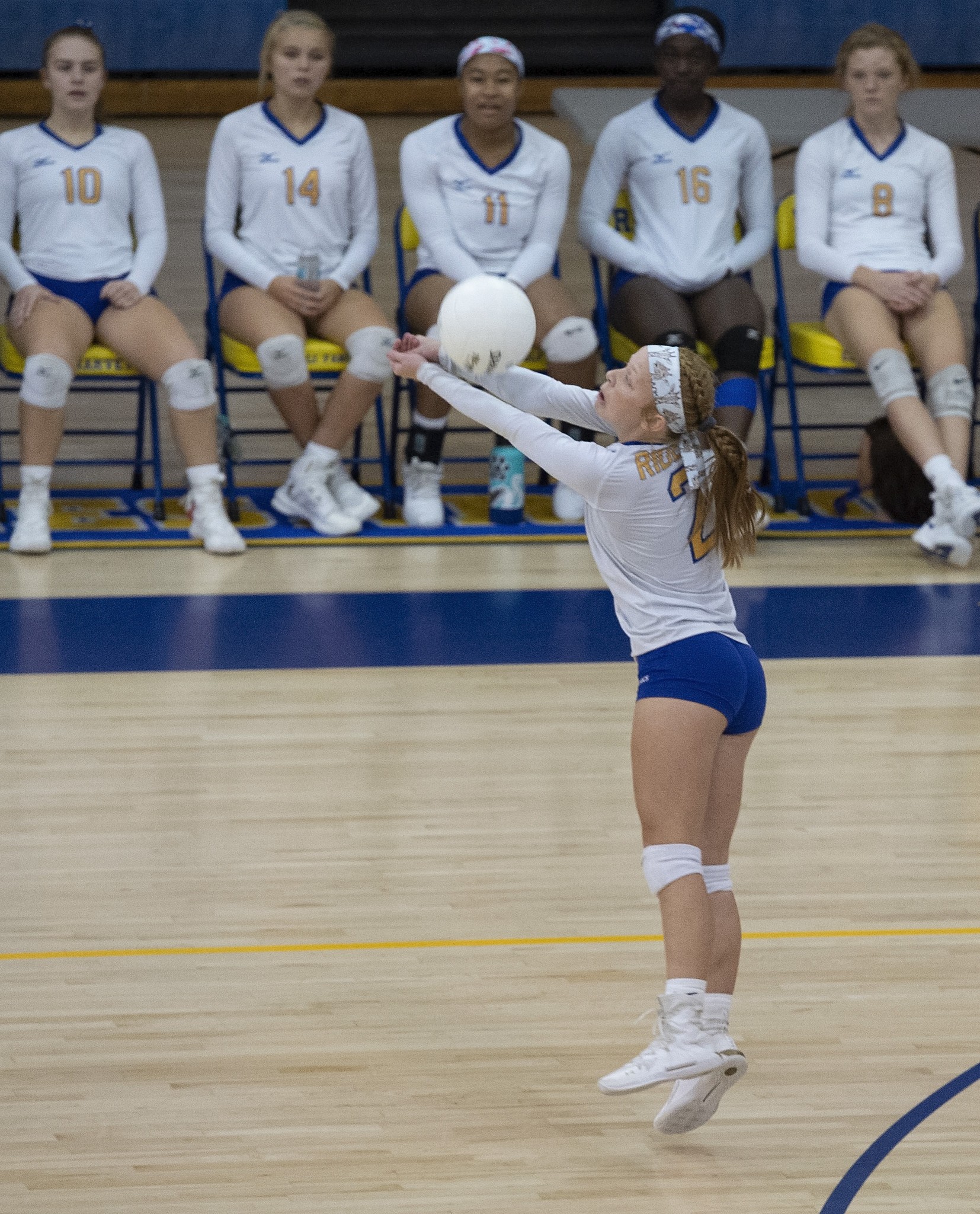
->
<box><xmin>910</xmin><ymin>481</ymin><xmax>980</xmax><ymax>567</ymax></box>
<box><xmin>552</xmin><ymin>482</ymin><xmax>586</xmax><ymax>521</ymax></box>
<box><xmin>400</xmin><ymin>457</ymin><xmax>446</xmax><ymax>529</ymax></box>
<box><xmin>9</xmin><ymin>483</ymin><xmax>54</xmax><ymax>554</ymax></box>
<box><xmin>595</xmin><ymin>988</ymin><xmax>727</xmax><ymax>1096</ymax></box>
<box><xmin>652</xmin><ymin>1028</ymin><xmax>748</xmax><ymax>1134</ymax></box>
<box><xmin>177</xmin><ymin>482</ymin><xmax>247</xmax><ymax>554</ymax></box>
<box><xmin>270</xmin><ymin>455</ymin><xmax>382</xmax><ymax>536</ymax></box>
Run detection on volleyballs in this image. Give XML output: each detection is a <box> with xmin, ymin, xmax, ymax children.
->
<box><xmin>435</xmin><ymin>272</ymin><xmax>538</xmax><ymax>378</ymax></box>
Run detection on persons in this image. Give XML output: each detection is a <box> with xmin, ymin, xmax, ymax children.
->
<box><xmin>576</xmin><ymin>7</ymin><xmax>777</xmax><ymax>442</ymax></box>
<box><xmin>387</xmin><ymin>331</ymin><xmax>769</xmax><ymax>1134</ymax></box>
<box><xmin>0</xmin><ymin>20</ymin><xmax>248</xmax><ymax>555</ymax></box>
<box><xmin>795</xmin><ymin>26</ymin><xmax>980</xmax><ymax>569</ymax></box>
<box><xmin>399</xmin><ymin>35</ymin><xmax>597</xmax><ymax>529</ymax></box>
<box><xmin>202</xmin><ymin>10</ymin><xmax>397</xmax><ymax>537</ymax></box>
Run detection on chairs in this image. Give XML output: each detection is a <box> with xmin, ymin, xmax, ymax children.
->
<box><xmin>771</xmin><ymin>191</ymin><xmax>920</xmax><ymax>514</ymax></box>
<box><xmin>584</xmin><ymin>186</ymin><xmax>785</xmax><ymax>514</ymax></box>
<box><xmin>200</xmin><ymin>213</ymin><xmax>394</xmax><ymax>521</ymax></box>
<box><xmin>0</xmin><ymin>282</ymin><xmax>167</xmax><ymax>519</ymax></box>
<box><xmin>389</xmin><ymin>201</ymin><xmax>562</xmax><ymax>500</ymax></box>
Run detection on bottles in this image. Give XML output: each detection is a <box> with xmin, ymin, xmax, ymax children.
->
<box><xmin>489</xmin><ymin>433</ymin><xmax>524</xmax><ymax>524</ymax></box>
